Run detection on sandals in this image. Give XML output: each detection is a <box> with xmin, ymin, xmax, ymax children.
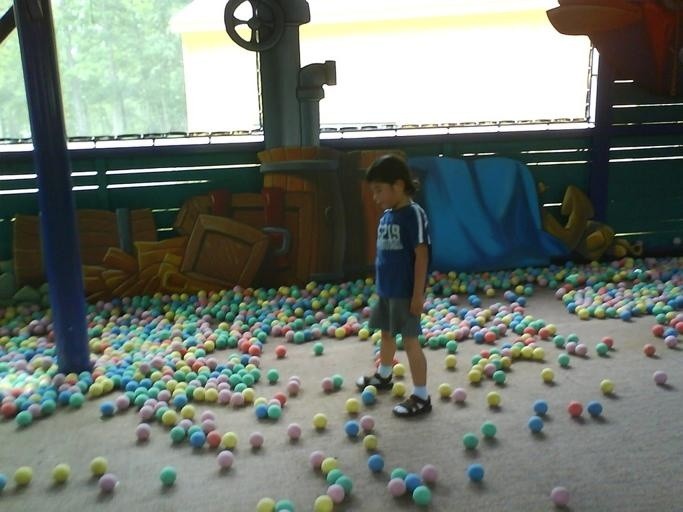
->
<box><xmin>355</xmin><ymin>371</ymin><xmax>395</xmax><ymax>392</ymax></box>
<box><xmin>392</xmin><ymin>393</ymin><xmax>434</xmax><ymax>418</ymax></box>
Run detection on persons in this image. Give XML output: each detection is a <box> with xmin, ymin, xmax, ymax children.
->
<box><xmin>357</xmin><ymin>152</ymin><xmax>434</xmax><ymax>417</ymax></box>
